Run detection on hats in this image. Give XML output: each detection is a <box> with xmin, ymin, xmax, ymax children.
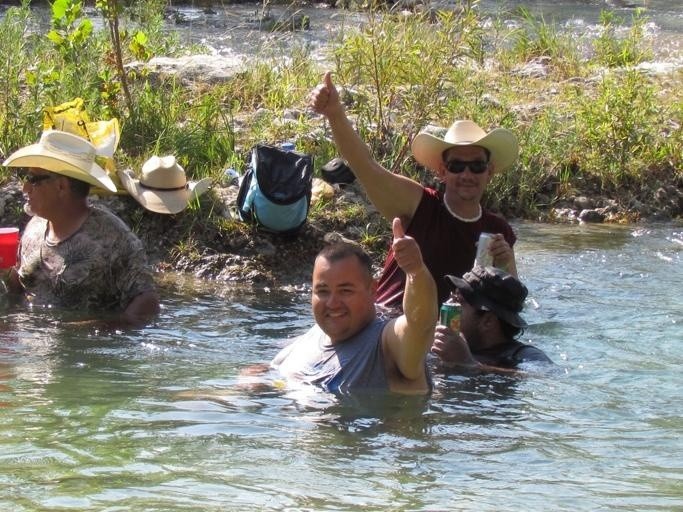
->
<box><xmin>443</xmin><ymin>264</ymin><xmax>528</xmax><ymax>328</ymax></box>
<box><xmin>0</xmin><ymin>130</ymin><xmax>118</xmax><ymax>194</ymax></box>
<box><xmin>411</xmin><ymin>119</ymin><xmax>519</xmax><ymax>173</ymax></box>
<box><xmin>116</xmin><ymin>154</ymin><xmax>212</xmax><ymax>215</ymax></box>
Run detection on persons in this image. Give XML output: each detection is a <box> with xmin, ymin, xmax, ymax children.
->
<box><xmin>428</xmin><ymin>264</ymin><xmax>562</xmax><ymax>376</ymax></box>
<box><xmin>310</xmin><ymin>69</ymin><xmax>518</xmax><ymax>315</ymax></box>
<box><xmin>0</xmin><ymin>131</ymin><xmax>161</xmax><ymax>327</ymax></box>
<box><xmin>236</xmin><ymin>214</ymin><xmax>440</xmax><ymax>394</ymax></box>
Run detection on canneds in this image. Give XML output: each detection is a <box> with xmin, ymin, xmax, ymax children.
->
<box><xmin>472</xmin><ymin>231</ymin><xmax>494</xmax><ymax>271</ymax></box>
<box><xmin>440</xmin><ymin>303</ymin><xmax>463</xmax><ymax>336</ymax></box>
<box><xmin>225</xmin><ymin>169</ymin><xmax>239</xmax><ymax>184</ymax></box>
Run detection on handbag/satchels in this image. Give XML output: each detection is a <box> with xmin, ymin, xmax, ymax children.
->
<box><xmin>320</xmin><ymin>157</ymin><xmax>355</xmax><ymax>187</ymax></box>
<box><xmin>41</xmin><ymin>97</ymin><xmax>120</xmax><ymax>154</ymax></box>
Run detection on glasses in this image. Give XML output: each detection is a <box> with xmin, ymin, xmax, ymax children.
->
<box><xmin>26</xmin><ymin>171</ymin><xmax>50</xmax><ymax>186</ymax></box>
<box><xmin>444</xmin><ymin>159</ymin><xmax>488</xmax><ymax>174</ymax></box>
<box><xmin>449</xmin><ymin>290</ymin><xmax>467</xmax><ymax>306</ymax></box>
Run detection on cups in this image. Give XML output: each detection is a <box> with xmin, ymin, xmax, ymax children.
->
<box><xmin>0</xmin><ymin>227</ymin><xmax>20</xmax><ymax>269</ymax></box>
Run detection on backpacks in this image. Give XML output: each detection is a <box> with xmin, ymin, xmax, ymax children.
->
<box><xmin>236</xmin><ymin>143</ymin><xmax>314</xmax><ymax>234</ymax></box>
